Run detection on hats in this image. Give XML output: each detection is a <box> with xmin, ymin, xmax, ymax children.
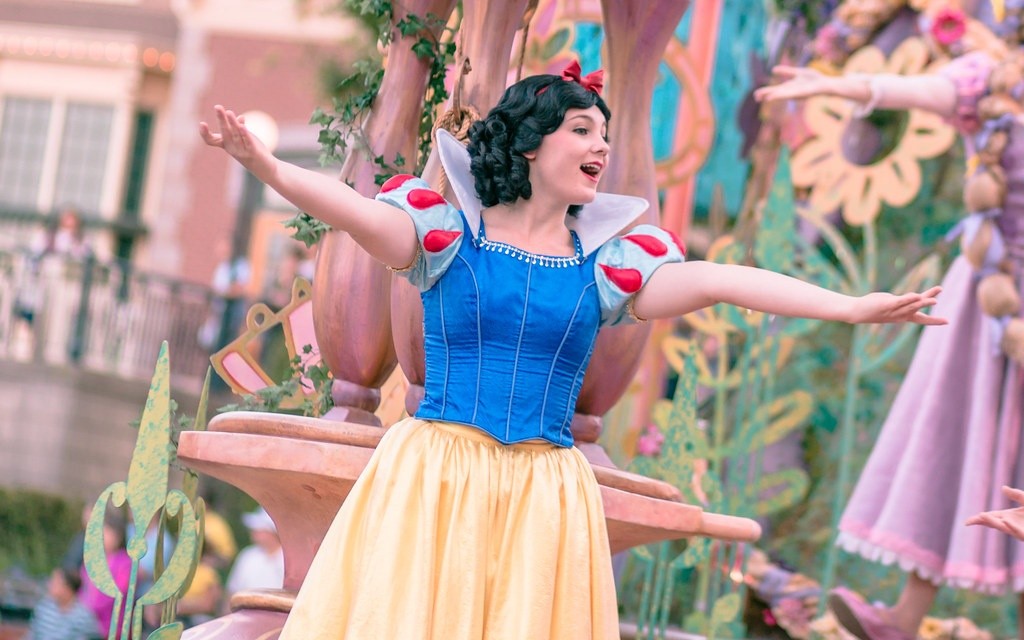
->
<box><xmin>242</xmin><ymin>508</ymin><xmax>278</xmax><ymax>534</ymax></box>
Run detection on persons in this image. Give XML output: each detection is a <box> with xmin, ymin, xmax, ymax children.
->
<box><xmin>197</xmin><ymin>223</ymin><xmax>256</xmax><ymax>397</ymax></box>
<box><xmin>199</xmin><ymin>60</ymin><xmax>949</xmax><ymax>640</ymax></box>
<box><xmin>253</xmin><ymin>241</ymin><xmax>311</xmax><ymax>385</ymax></box>
<box><xmin>26</xmin><ymin>482</ymin><xmax>285</xmax><ymax>639</ymax></box>
<box><xmin>6</xmin><ymin>221</ymin><xmax>56</xmax><ymax>362</ymax></box>
<box><xmin>753</xmin><ymin>35</ymin><xmax>1024</xmax><ymax>640</ymax></box>
<box><xmin>964</xmin><ymin>485</ymin><xmax>1024</xmax><ymax>543</ymax></box>
<box><xmin>51</xmin><ymin>209</ymin><xmax>95</xmax><ymax>362</ymax></box>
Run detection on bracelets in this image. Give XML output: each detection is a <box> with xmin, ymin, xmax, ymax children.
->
<box><xmin>851</xmin><ymin>72</ymin><xmax>882</xmax><ymax>120</ymax></box>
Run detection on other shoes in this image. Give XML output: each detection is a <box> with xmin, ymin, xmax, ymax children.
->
<box><xmin>829</xmin><ymin>586</ymin><xmax>919</xmax><ymax>640</ymax></box>
